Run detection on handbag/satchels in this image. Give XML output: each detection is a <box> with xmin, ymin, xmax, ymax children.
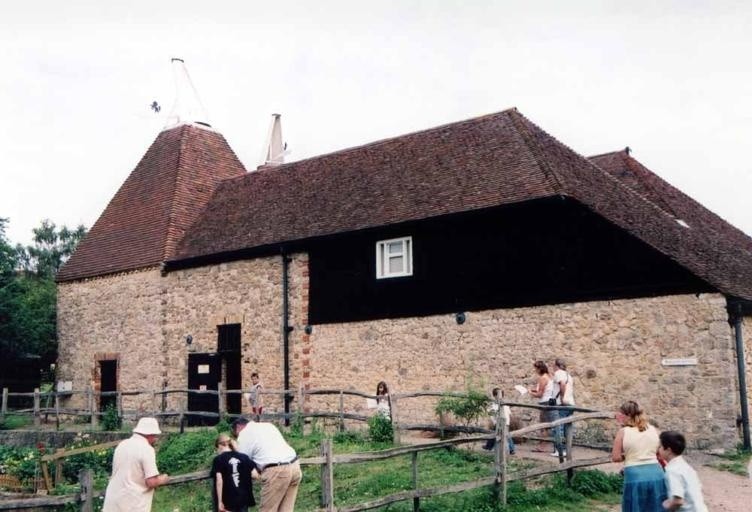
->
<box><xmin>549</xmin><ymin>397</ymin><xmax>557</xmax><ymax>406</ymax></box>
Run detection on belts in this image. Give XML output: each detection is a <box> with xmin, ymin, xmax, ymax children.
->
<box><xmin>265</xmin><ymin>456</ymin><xmax>297</xmax><ymax>469</ymax></box>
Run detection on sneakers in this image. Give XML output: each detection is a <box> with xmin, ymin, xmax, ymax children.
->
<box><xmin>551</xmin><ymin>450</ymin><xmax>567</xmax><ymax>457</ymax></box>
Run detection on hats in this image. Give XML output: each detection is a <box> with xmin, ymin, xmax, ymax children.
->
<box><xmin>134</xmin><ymin>417</ymin><xmax>161</xmax><ymax>435</ymax></box>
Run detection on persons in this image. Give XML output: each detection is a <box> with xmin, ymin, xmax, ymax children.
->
<box><xmin>102</xmin><ymin>417</ymin><xmax>170</xmax><ymax>512</ymax></box>
<box><xmin>611</xmin><ymin>401</ymin><xmax>668</xmax><ymax>512</ymax></box>
<box><xmin>528</xmin><ymin>360</ymin><xmax>553</xmax><ymax>452</ymax></box>
<box><xmin>482</xmin><ymin>387</ymin><xmax>516</xmax><ymax>454</ymax></box>
<box><xmin>230</xmin><ymin>417</ymin><xmax>303</xmax><ymax>512</ymax></box>
<box><xmin>248</xmin><ymin>372</ymin><xmax>264</xmax><ymax>422</ymax></box>
<box><xmin>209</xmin><ymin>434</ymin><xmax>259</xmax><ymax>512</ymax></box>
<box><xmin>658</xmin><ymin>431</ymin><xmax>708</xmax><ymax>512</ymax></box>
<box><xmin>376</xmin><ymin>381</ymin><xmax>393</xmax><ymax>423</ymax></box>
<box><xmin>549</xmin><ymin>358</ymin><xmax>575</xmax><ymax>458</ymax></box>
